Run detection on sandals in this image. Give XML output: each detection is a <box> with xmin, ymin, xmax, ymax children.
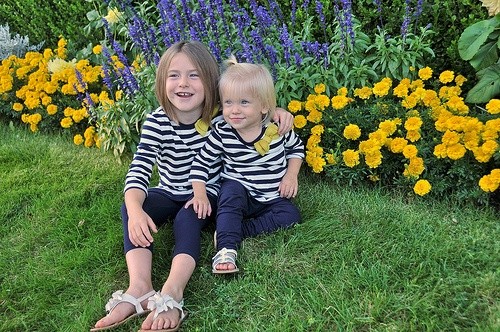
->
<box><xmin>139</xmin><ymin>292</ymin><xmax>190</xmax><ymax>332</ymax></box>
<box><xmin>211</xmin><ymin>248</ymin><xmax>239</xmax><ymax>273</ymax></box>
<box><xmin>89</xmin><ymin>289</ymin><xmax>162</xmax><ymax>332</ymax></box>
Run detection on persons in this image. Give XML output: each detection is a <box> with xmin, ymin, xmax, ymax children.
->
<box><xmin>184</xmin><ymin>52</ymin><xmax>307</xmax><ymax>274</ymax></box>
<box><xmin>90</xmin><ymin>41</ymin><xmax>294</xmax><ymax>332</ymax></box>
